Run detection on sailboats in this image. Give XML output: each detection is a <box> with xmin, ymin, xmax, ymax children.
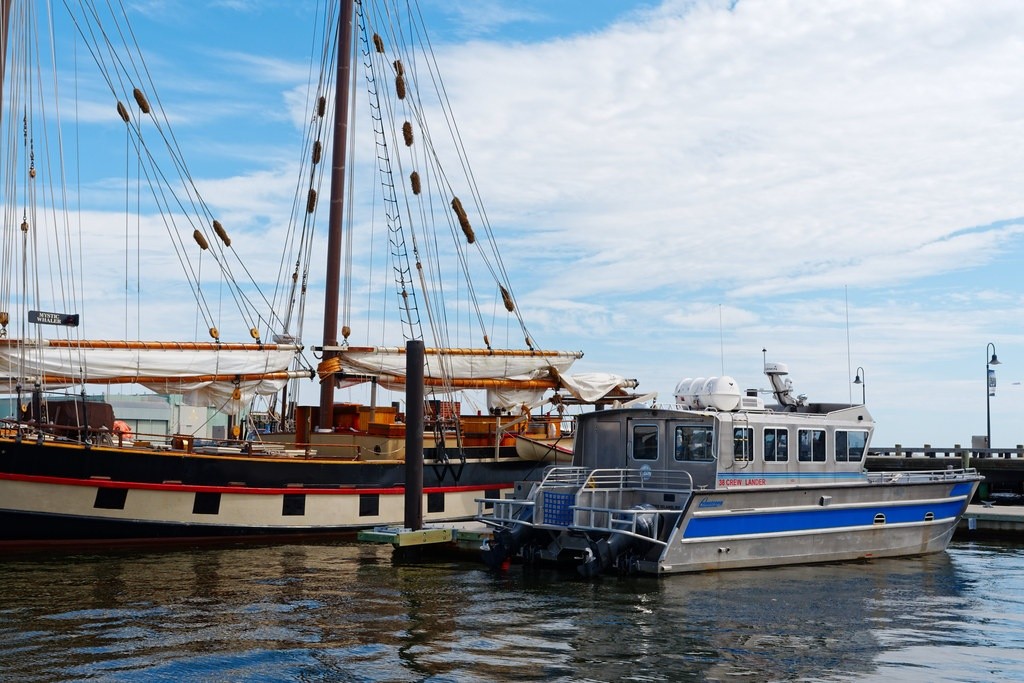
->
<box><xmin>0</xmin><ymin>0</ymin><xmax>657</xmax><ymax>541</ymax></box>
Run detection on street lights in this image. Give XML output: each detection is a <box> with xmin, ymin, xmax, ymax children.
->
<box><xmin>985</xmin><ymin>342</ymin><xmax>1003</xmax><ymax>458</ymax></box>
<box><xmin>853</xmin><ymin>366</ymin><xmax>866</xmax><ymax>405</ymax></box>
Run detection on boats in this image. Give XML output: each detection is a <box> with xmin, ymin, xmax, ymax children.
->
<box><xmin>472</xmin><ymin>283</ymin><xmax>988</xmax><ymax>580</ymax></box>
<box><xmin>515</xmin><ymin>434</ymin><xmax>575</xmax><ymax>463</ymax></box>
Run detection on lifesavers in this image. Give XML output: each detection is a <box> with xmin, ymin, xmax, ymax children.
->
<box><xmin>113</xmin><ymin>420</ymin><xmax>133</xmax><ymax>437</ymax></box>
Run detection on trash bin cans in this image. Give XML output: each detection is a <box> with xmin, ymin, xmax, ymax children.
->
<box><xmin>971</xmin><ymin>482</ymin><xmax>989</xmax><ymax>505</ymax></box>
